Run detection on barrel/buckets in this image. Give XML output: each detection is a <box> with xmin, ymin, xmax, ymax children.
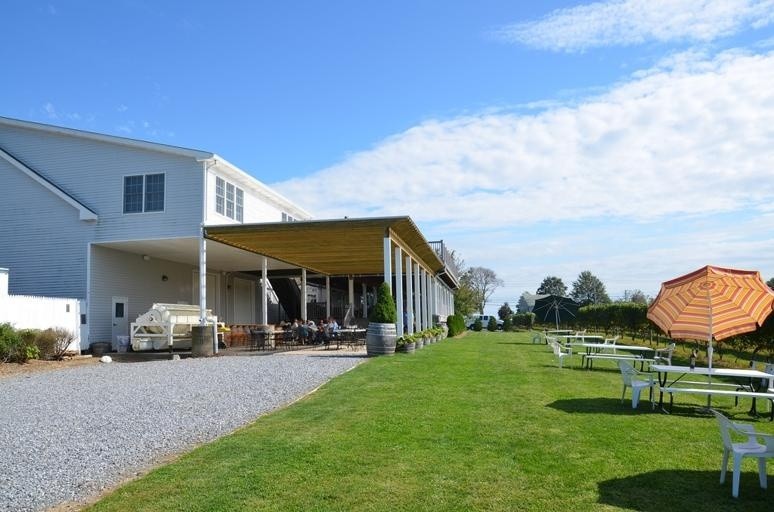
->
<box><xmin>257</xmin><ymin>324</ymin><xmax>262</xmax><ymax>330</ymax></box>
<box><xmin>230</xmin><ymin>324</ymin><xmax>238</xmax><ymax>347</ymax></box>
<box><xmin>218</xmin><ymin>323</ymin><xmax>225</xmax><ymax>341</ymax></box>
<box><xmin>224</xmin><ymin>324</ymin><xmax>232</xmax><ymax>347</ymax></box>
<box><xmin>243</xmin><ymin>325</ymin><xmax>251</xmax><ymax>347</ymax></box>
<box><xmin>237</xmin><ymin>324</ymin><xmax>245</xmax><ymax>347</ymax></box>
<box><xmin>249</xmin><ymin>323</ymin><xmax>257</xmax><ymax>332</ymax></box>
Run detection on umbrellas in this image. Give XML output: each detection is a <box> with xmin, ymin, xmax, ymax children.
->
<box><xmin>647</xmin><ymin>265</ymin><xmax>774</xmax><ymax>408</ymax></box>
<box><xmin>532</xmin><ymin>295</ymin><xmax>580</xmax><ymax>336</ymax></box>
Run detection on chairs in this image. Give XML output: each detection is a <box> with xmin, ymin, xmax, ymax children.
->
<box><xmin>246</xmin><ymin>325</ymin><xmax>366</xmax><ymax>352</ymax></box>
<box><xmin>530</xmin><ymin>330</ymin><xmax>774</xmax><ymax>421</ymax></box>
<box><xmin>710</xmin><ymin>409</ymin><xmax>774</xmax><ymax>498</ymax></box>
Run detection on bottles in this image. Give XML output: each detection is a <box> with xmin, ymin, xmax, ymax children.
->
<box><xmin>689</xmin><ymin>349</ymin><xmax>697</xmax><ymax>368</ymax></box>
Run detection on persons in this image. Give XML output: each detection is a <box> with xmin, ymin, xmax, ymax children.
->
<box><xmin>283</xmin><ymin>318</ymin><xmax>338</xmax><ymax>345</ymax></box>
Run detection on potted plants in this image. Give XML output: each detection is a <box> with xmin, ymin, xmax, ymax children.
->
<box><xmin>366</xmin><ymin>282</ymin><xmax>447</xmax><ymax>356</ymax></box>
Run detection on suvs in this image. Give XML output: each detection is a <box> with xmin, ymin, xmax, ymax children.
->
<box><xmin>466</xmin><ymin>314</ymin><xmax>504</xmax><ymax>330</ymax></box>
